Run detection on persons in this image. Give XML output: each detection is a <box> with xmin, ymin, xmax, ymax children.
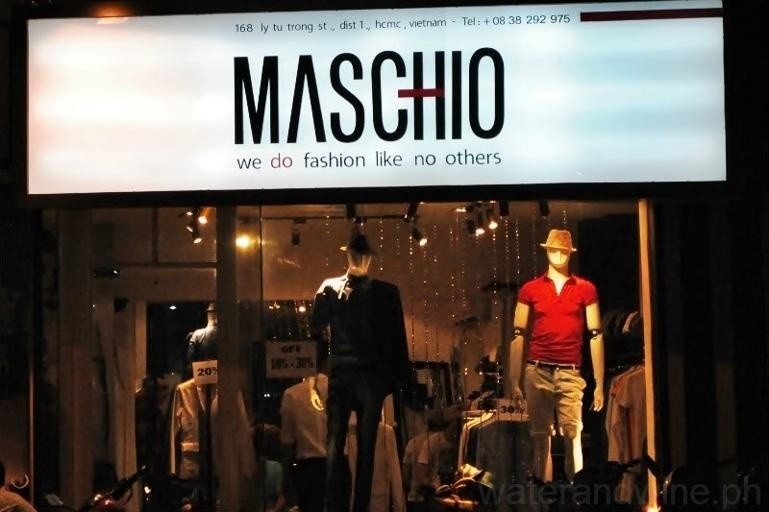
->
<box><xmin>184</xmin><ymin>301</ymin><xmax>219</xmax><ymax>364</ymax></box>
<box><xmin>279</xmin><ymin>372</ymin><xmax>355</xmax><ymax>512</ymax></box>
<box><xmin>0</xmin><ymin>462</ymin><xmax>37</xmax><ymax>512</ymax></box>
<box><xmin>416</xmin><ymin>406</ymin><xmax>463</xmax><ymax>490</ymax></box>
<box><xmin>402</xmin><ymin>407</ymin><xmax>445</xmax><ymax>500</ymax></box>
<box><xmin>506</xmin><ymin>228</ymin><xmax>605</xmax><ymax>484</ymax></box>
<box><xmin>304</xmin><ymin>233</ymin><xmax>408</xmax><ymax>510</ymax></box>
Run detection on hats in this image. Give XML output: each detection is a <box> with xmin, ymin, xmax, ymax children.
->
<box><xmin>339</xmin><ymin>232</ymin><xmax>377</xmax><ymax>258</ymax></box>
<box><xmin>539</xmin><ymin>227</ymin><xmax>579</xmax><ymax>254</ymax></box>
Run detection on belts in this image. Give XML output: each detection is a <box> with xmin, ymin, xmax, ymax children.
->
<box><xmin>527</xmin><ymin>360</ymin><xmax>583</xmax><ymax>371</ymax></box>
